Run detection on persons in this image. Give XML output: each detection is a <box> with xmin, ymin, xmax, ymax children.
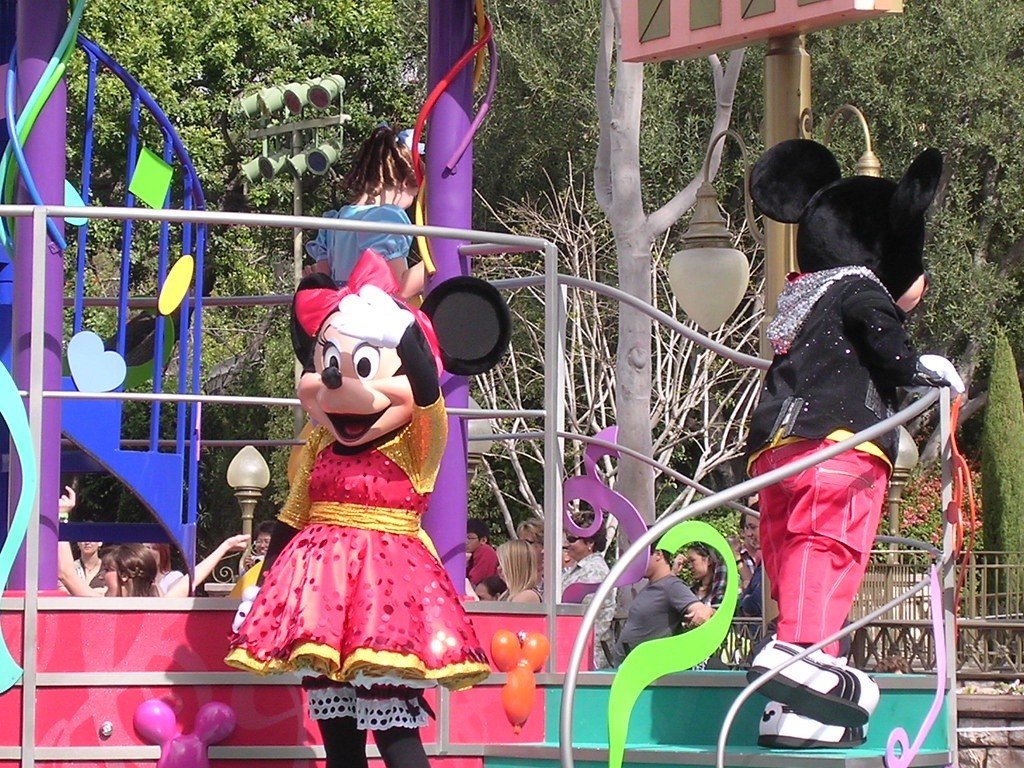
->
<box><xmin>607</xmin><ymin>498</ymin><xmax>767</xmax><ymax>670</ymax></box>
<box><xmin>462</xmin><ymin>512</ymin><xmax>610</xmax><ymax>622</ymax></box>
<box><xmin>284</xmin><ymin>122</ymin><xmax>427</xmax><ymax>308</ymax></box>
<box><xmin>57</xmin><ymin>484</ymin><xmax>274</xmax><ymax>597</ymax></box>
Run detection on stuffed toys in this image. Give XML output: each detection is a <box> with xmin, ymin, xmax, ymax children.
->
<box><xmin>744</xmin><ymin>138</ymin><xmax>964</xmax><ymax>748</ymax></box>
<box><xmin>222</xmin><ymin>248</ymin><xmax>512</xmax><ymax>768</ymax></box>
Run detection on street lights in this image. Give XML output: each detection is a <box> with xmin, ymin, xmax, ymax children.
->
<box><xmin>884</xmin><ymin>425</ymin><xmax>919</xmax><ymax>656</ymax></box>
<box><xmin>666</xmin><ymin>102</ymin><xmax>880</xmax><ymax>631</ymax></box>
<box><xmin>212</xmin><ymin>444</ymin><xmax>271</xmax><ymax>584</ymax></box>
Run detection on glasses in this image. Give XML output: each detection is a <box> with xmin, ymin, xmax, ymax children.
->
<box><xmin>566</xmin><ymin>536</ymin><xmax>586</xmax><ymax>543</ymax></box>
<box><xmin>693</xmin><ymin>541</ymin><xmax>708</xmax><ymax>551</ymax></box>
<box><xmin>253</xmin><ymin>540</ymin><xmax>271</xmax><ymax>546</ymax></box>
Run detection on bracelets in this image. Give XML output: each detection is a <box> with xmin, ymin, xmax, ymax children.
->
<box><xmin>736</xmin><ymin>559</ymin><xmax>743</xmax><ymax>564</ymax></box>
<box><xmin>59</xmin><ymin>512</ymin><xmax>68</xmax><ymax>518</ymax></box>
<box><xmin>59</xmin><ymin>519</ymin><xmax>69</xmax><ymax>523</ymax></box>
